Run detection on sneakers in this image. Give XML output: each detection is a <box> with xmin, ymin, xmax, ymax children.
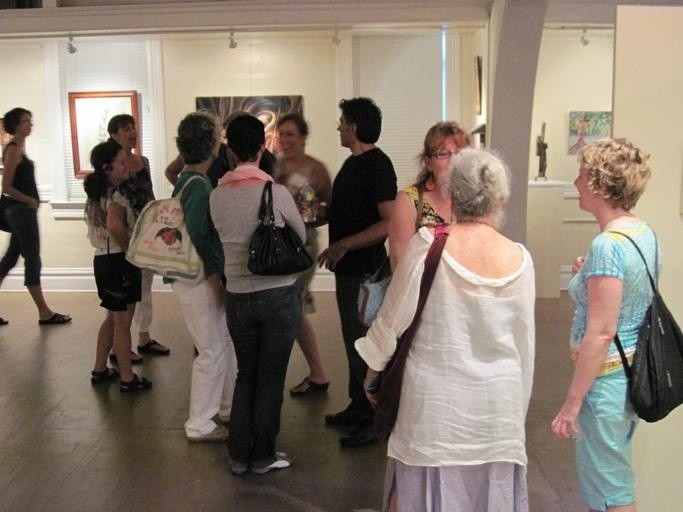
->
<box><xmin>227</xmin><ymin>457</ymin><xmax>248</xmax><ymax>474</ymax></box>
<box><xmin>217</xmin><ymin>412</ymin><xmax>231</xmax><ymax>422</ymax></box>
<box><xmin>186</xmin><ymin>425</ymin><xmax>229</xmax><ymax>444</ymax></box>
<box><xmin>251</xmin><ymin>456</ymin><xmax>293</xmax><ymax>474</ymax></box>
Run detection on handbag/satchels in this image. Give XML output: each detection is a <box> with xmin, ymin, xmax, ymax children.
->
<box><xmin>368</xmin><ymin>229</ymin><xmax>448</xmax><ymax>415</ymax></box>
<box><xmin>0</xmin><ymin>142</ymin><xmax>20</xmax><ymax>234</ymax></box>
<box><xmin>247</xmin><ymin>179</ymin><xmax>313</xmax><ymax>276</ymax></box>
<box><xmin>125</xmin><ymin>176</ymin><xmax>209</xmax><ymax>286</ymax></box>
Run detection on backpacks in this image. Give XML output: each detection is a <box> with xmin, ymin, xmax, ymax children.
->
<box><xmin>608</xmin><ymin>224</ymin><xmax>683</xmax><ymax>423</ymax></box>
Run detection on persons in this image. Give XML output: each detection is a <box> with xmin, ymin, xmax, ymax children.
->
<box><xmin>274</xmin><ymin>111</ymin><xmax>332</xmax><ymax>397</ymax></box>
<box><xmin>0</xmin><ymin>107</ymin><xmax>73</xmax><ymax>327</ymax></box>
<box><xmin>83</xmin><ymin>141</ymin><xmax>154</xmax><ymax>391</ymax></box>
<box><xmin>298</xmin><ymin>96</ymin><xmax>398</xmax><ymax>451</ymax></box>
<box><xmin>163</xmin><ymin>112</ymin><xmax>239</xmax><ymax>443</ymax></box>
<box><xmin>549</xmin><ymin>137</ymin><xmax>662</xmax><ymax>511</ymax></box>
<box><xmin>354</xmin><ymin>145</ymin><xmax>529</xmax><ymax>512</ymax></box>
<box><xmin>107</xmin><ymin>113</ymin><xmax>170</xmax><ymax>365</ymax></box>
<box><xmin>165</xmin><ymin>108</ymin><xmax>228</xmax><ymax>193</ymax></box>
<box><xmin>387</xmin><ymin>120</ymin><xmax>473</xmax><ymax>276</ymax></box>
<box><xmin>209</xmin><ymin>113</ymin><xmax>308</xmax><ymax>474</ymax></box>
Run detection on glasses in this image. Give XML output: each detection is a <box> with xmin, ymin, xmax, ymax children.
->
<box><xmin>427</xmin><ymin>150</ymin><xmax>458</xmax><ymax>160</ymax></box>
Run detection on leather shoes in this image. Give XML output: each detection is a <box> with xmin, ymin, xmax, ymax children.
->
<box><xmin>324</xmin><ymin>399</ymin><xmax>368</xmax><ymax>427</ymax></box>
<box><xmin>338</xmin><ymin>423</ymin><xmax>378</xmax><ymax>447</ymax></box>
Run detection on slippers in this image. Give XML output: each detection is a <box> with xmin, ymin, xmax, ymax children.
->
<box><xmin>37</xmin><ymin>313</ymin><xmax>72</xmax><ymax>324</ymax></box>
<box><xmin>0</xmin><ymin>316</ymin><xmax>9</xmax><ymax>326</ymax></box>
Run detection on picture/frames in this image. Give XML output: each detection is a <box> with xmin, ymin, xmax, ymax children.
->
<box><xmin>0</xmin><ymin>117</ymin><xmax>14</xmax><ymax>174</ymax></box>
<box><xmin>568</xmin><ymin>111</ymin><xmax>612</xmax><ymax>154</ymax></box>
<box><xmin>68</xmin><ymin>91</ymin><xmax>141</xmax><ymax>180</ymax></box>
<box><xmin>196</xmin><ymin>96</ymin><xmax>305</xmax><ymax>158</ymax></box>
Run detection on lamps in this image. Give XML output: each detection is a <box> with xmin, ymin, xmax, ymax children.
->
<box><xmin>578</xmin><ymin>32</ymin><xmax>590</xmax><ymax>48</ymax></box>
<box><xmin>332</xmin><ymin>34</ymin><xmax>343</xmax><ymax>47</ymax></box>
<box><xmin>227</xmin><ymin>36</ymin><xmax>238</xmax><ymax>50</ymax></box>
<box><xmin>66</xmin><ymin>42</ymin><xmax>77</xmax><ymax>54</ymax></box>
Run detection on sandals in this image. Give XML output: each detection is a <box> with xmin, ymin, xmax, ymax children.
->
<box><xmin>119</xmin><ymin>373</ymin><xmax>152</xmax><ymax>393</ymax></box>
<box><xmin>289</xmin><ymin>376</ymin><xmax>329</xmax><ymax>397</ymax></box>
<box><xmin>137</xmin><ymin>339</ymin><xmax>170</xmax><ymax>355</ymax></box>
<box><xmin>90</xmin><ymin>366</ymin><xmax>119</xmax><ymax>384</ymax></box>
<box><xmin>109</xmin><ymin>352</ymin><xmax>143</xmax><ymax>363</ymax></box>
